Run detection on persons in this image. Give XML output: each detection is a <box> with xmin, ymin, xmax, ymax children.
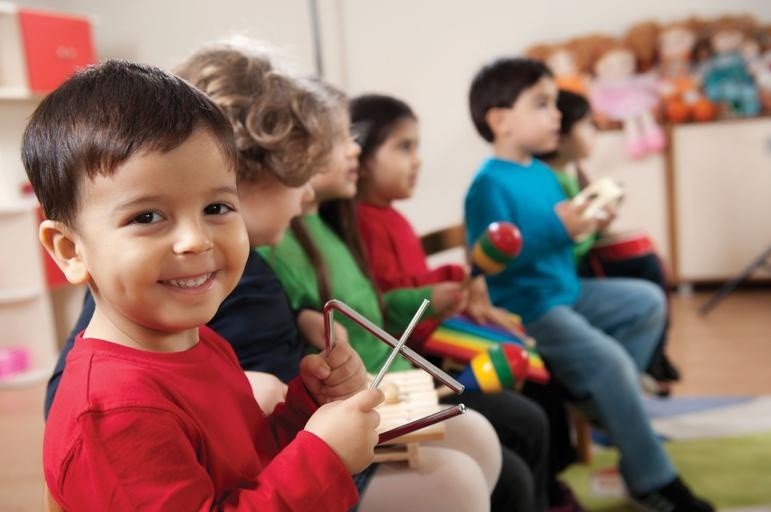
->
<box><xmin>21</xmin><ymin>59</ymin><xmax>382</xmax><ymax>511</ymax></box>
<box><xmin>462</xmin><ymin>58</ymin><xmax>717</xmax><ymax>510</ymax></box>
<box><xmin>45</xmin><ymin>48</ymin><xmax>504</xmax><ymax>512</ymax></box>
<box><xmin>526</xmin><ymin>41</ymin><xmax>605</xmax><ymax>130</ymax></box>
<box><xmin>259</xmin><ymin>77</ymin><xmax>550</xmax><ymax>511</ymax></box>
<box><xmin>534</xmin><ymin>91</ymin><xmax>680</xmax><ymax>397</ymax></box>
<box><xmin>641</xmin><ymin>16</ymin><xmax>717</xmax><ymax>121</ymax></box>
<box><xmin>349</xmin><ymin>94</ymin><xmax>579</xmax><ymax>472</ymax></box>
<box><xmin>591</xmin><ymin>39</ymin><xmax>667</xmax><ymax>157</ymax></box>
<box><xmin>692</xmin><ymin>16</ymin><xmax>771</xmax><ymax>116</ymax></box>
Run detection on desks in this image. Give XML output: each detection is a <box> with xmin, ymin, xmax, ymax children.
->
<box><xmin>561</xmin><ymin>115</ymin><xmax>769</xmax><ymax>290</ymax></box>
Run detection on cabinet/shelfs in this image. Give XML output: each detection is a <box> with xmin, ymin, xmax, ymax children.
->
<box><xmin>1</xmin><ymin>13</ymin><xmax>91</xmax><ymax>385</ymax></box>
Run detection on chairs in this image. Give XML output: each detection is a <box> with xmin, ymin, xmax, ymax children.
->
<box><xmin>419</xmin><ymin>222</ymin><xmax>466</xmax><ymax>263</ymax></box>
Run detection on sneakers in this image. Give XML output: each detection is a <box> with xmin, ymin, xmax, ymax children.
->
<box><xmin>644</xmin><ymin>352</ymin><xmax>680</xmax><ymax>382</ymax></box>
<box><xmin>620</xmin><ymin>477</ymin><xmax>716</xmax><ymax>511</ymax></box>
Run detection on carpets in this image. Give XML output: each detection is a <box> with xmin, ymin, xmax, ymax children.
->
<box><xmin>538</xmin><ymin>395</ymin><xmax>771</xmax><ymax>510</ymax></box>
